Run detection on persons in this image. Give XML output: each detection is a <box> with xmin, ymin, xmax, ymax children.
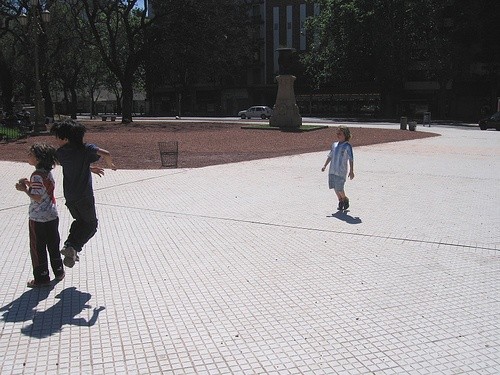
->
<box><xmin>53</xmin><ymin>119</ymin><xmax>117</xmax><ymax>268</ymax></box>
<box><xmin>15</xmin><ymin>143</ymin><xmax>66</xmax><ymax>288</ymax></box>
<box><xmin>321</xmin><ymin>125</ymin><xmax>354</xmax><ymax>210</ymax></box>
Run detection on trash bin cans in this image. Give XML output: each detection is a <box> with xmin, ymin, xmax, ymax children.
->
<box><xmin>158</xmin><ymin>141</ymin><xmax>178</xmax><ymax>166</ymax></box>
<box><xmin>424</xmin><ymin>112</ymin><xmax>431</xmax><ymax>122</ymax></box>
<box><xmin>400</xmin><ymin>117</ymin><xmax>407</xmax><ymax>130</ymax></box>
<box><xmin>409</xmin><ymin>122</ymin><xmax>417</xmax><ymax>131</ymax></box>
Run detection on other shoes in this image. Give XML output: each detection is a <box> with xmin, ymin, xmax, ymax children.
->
<box><xmin>338</xmin><ymin>202</ymin><xmax>342</xmax><ymax>210</ymax></box>
<box><xmin>27</xmin><ymin>278</ymin><xmax>51</xmax><ymax>287</ymax></box>
<box><xmin>64</xmin><ymin>246</ymin><xmax>76</xmax><ymax>268</ymax></box>
<box><xmin>60</xmin><ymin>248</ymin><xmax>79</xmax><ymax>261</ymax></box>
<box><xmin>55</xmin><ymin>272</ymin><xmax>65</xmax><ymax>280</ymax></box>
<box><xmin>342</xmin><ymin>198</ymin><xmax>349</xmax><ymax>209</ymax></box>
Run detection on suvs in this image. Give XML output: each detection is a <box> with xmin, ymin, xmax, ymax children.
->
<box><xmin>238</xmin><ymin>105</ymin><xmax>273</xmax><ymax>120</ymax></box>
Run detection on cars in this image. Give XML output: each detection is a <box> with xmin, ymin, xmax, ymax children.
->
<box><xmin>478</xmin><ymin>112</ymin><xmax>500</xmax><ymax>132</ymax></box>
